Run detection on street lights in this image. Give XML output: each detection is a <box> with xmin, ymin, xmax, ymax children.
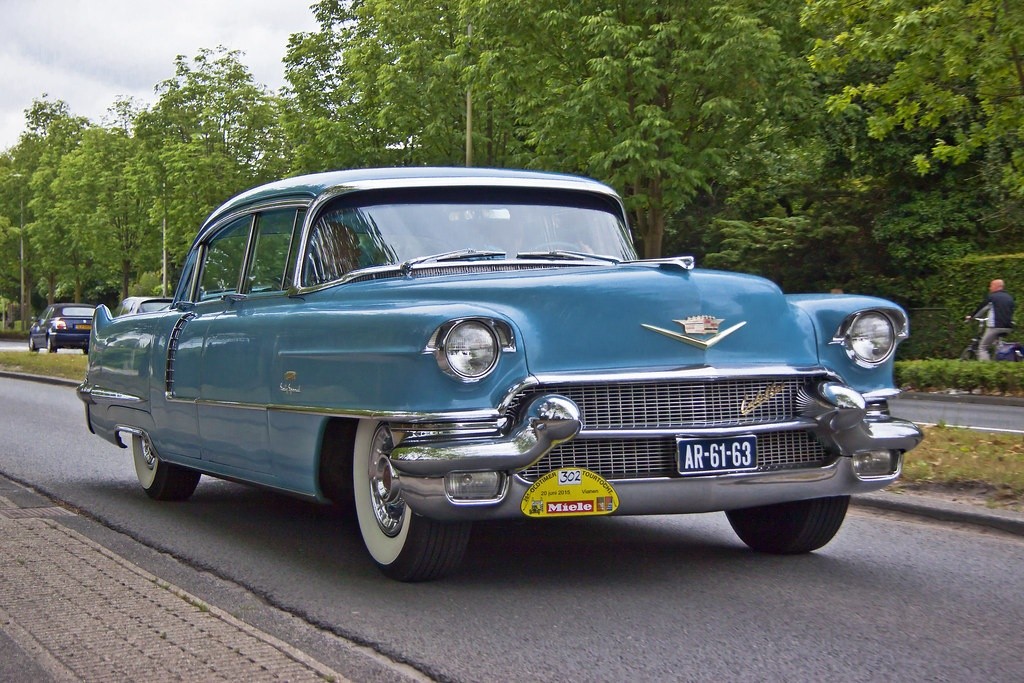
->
<box><xmin>12</xmin><ymin>173</ymin><xmax>27</xmax><ymax>331</ymax></box>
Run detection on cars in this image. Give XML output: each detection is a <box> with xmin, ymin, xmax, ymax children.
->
<box><xmin>28</xmin><ymin>302</ymin><xmax>98</xmax><ymax>355</ymax></box>
<box><xmin>117</xmin><ymin>295</ymin><xmax>175</xmax><ymax>319</ymax></box>
<box><xmin>81</xmin><ymin>167</ymin><xmax>926</xmax><ymax>584</ymax></box>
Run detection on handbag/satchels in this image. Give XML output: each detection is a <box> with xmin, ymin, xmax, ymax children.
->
<box><xmin>995</xmin><ymin>343</ymin><xmax>1024</xmax><ymax>361</ymax></box>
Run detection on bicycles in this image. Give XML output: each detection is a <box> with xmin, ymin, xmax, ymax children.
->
<box><xmin>960</xmin><ymin>315</ymin><xmax>1024</xmax><ymax>363</ymax></box>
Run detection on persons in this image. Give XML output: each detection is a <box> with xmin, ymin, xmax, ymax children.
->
<box><xmin>271</xmin><ymin>224</ymin><xmax>373</xmax><ymax>291</ymax></box>
<box><xmin>965</xmin><ymin>279</ymin><xmax>1014</xmax><ymax>362</ymax></box>
